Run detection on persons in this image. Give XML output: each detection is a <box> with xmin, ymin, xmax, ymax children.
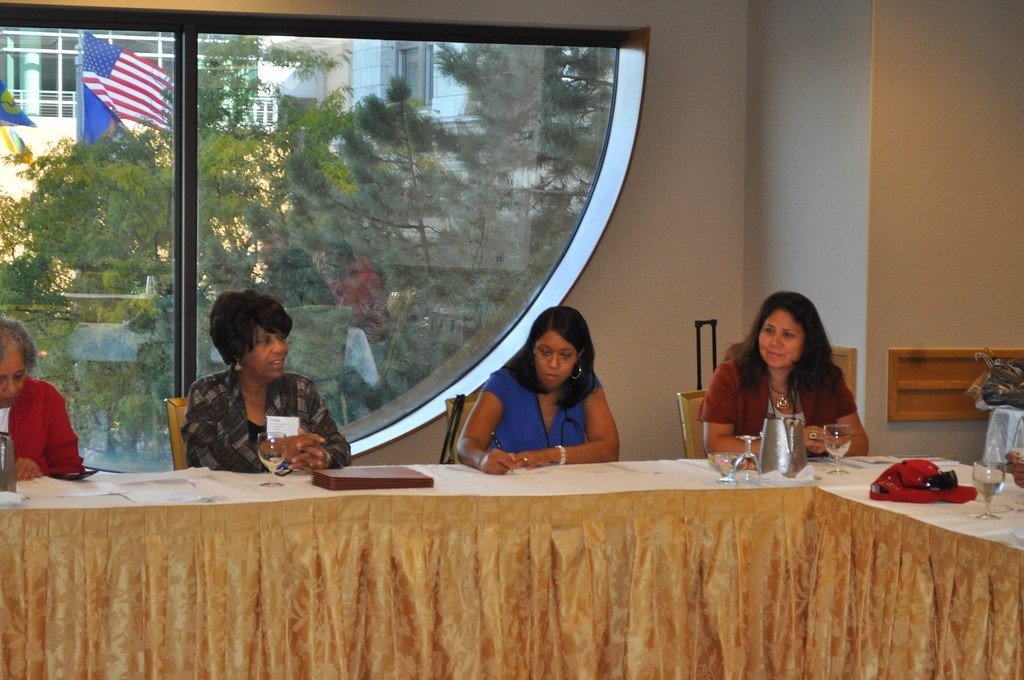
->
<box><xmin>1005</xmin><ymin>452</ymin><xmax>1024</xmax><ymax>489</ymax></box>
<box><xmin>457</xmin><ymin>306</ymin><xmax>619</xmax><ymax>475</ymax></box>
<box><xmin>179</xmin><ymin>287</ymin><xmax>351</xmax><ymax>474</ymax></box>
<box><xmin>0</xmin><ymin>313</ymin><xmax>86</xmax><ymax>481</ymax></box>
<box><xmin>697</xmin><ymin>290</ymin><xmax>869</xmax><ymax>461</ymax></box>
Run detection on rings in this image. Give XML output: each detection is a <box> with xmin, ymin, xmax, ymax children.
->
<box><xmin>523</xmin><ymin>457</ymin><xmax>528</xmax><ymax>467</ymax></box>
<box><xmin>808</xmin><ymin>432</ymin><xmax>818</xmax><ymax>439</ymax></box>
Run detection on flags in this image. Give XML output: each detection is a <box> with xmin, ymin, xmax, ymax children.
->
<box><xmin>82</xmin><ymin>30</ymin><xmax>172</xmax><ymax>134</ymax></box>
<box><xmin>83</xmin><ymin>82</ymin><xmax>132</xmax><ymax>142</ymax></box>
<box><xmin>0</xmin><ymin>78</ymin><xmax>37</xmax><ymax>127</ymax></box>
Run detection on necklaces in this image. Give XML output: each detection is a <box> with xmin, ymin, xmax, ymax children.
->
<box><xmin>769</xmin><ymin>384</ymin><xmax>790</xmax><ymax>410</ymax></box>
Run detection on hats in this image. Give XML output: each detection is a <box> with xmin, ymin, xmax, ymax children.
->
<box><xmin>869</xmin><ymin>459</ymin><xmax>977</xmax><ymax>504</ymax></box>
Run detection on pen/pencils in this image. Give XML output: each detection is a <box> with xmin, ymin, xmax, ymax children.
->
<box><xmin>491</xmin><ymin>432</ymin><xmax>514</xmax><ymax>474</ymax></box>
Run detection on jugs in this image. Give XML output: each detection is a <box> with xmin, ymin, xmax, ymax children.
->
<box><xmin>0</xmin><ymin>431</ymin><xmax>17</xmax><ymax>493</ymax></box>
<box><xmin>758</xmin><ymin>417</ymin><xmax>807</xmax><ymax>479</ymax></box>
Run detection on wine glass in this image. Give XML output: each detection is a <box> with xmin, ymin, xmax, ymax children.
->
<box><xmin>256</xmin><ymin>432</ymin><xmax>287</xmax><ymax>488</ymax></box>
<box><xmin>823</xmin><ymin>423</ymin><xmax>851</xmax><ymax>478</ymax></box>
<box><xmin>972</xmin><ymin>460</ymin><xmax>1006</xmax><ymax>522</ymax></box>
<box><xmin>730</xmin><ymin>435</ymin><xmax>762</xmax><ymax>485</ymax></box>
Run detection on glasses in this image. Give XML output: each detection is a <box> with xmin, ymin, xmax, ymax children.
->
<box><xmin>531</xmin><ymin>344</ymin><xmax>585</xmax><ymax>365</ymax></box>
<box><xmin>897</xmin><ymin>470</ymin><xmax>959</xmax><ymax>491</ymax></box>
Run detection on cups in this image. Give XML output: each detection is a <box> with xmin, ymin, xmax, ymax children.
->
<box><xmin>708</xmin><ymin>452</ymin><xmax>741</xmax><ymax>476</ymax></box>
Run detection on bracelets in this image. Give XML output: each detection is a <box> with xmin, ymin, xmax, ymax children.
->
<box><xmin>555</xmin><ymin>445</ymin><xmax>566</xmax><ymax>465</ymax></box>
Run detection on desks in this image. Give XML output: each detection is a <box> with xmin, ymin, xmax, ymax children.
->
<box><xmin>65</xmin><ymin>321</ymin><xmax>381</xmax><ymax>388</ymax></box>
<box><xmin>0</xmin><ymin>456</ymin><xmax>1024</xmax><ymax>680</ymax></box>
<box><xmin>983</xmin><ymin>407</ymin><xmax>1024</xmax><ymax>466</ymax></box>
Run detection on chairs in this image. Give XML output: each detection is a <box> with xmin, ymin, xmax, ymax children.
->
<box><xmin>384</xmin><ymin>287</ymin><xmax>413</xmax><ymax>373</ymax></box>
<box><xmin>285</xmin><ymin>305</ymin><xmax>351</xmax><ymax>425</ymax></box>
<box><xmin>166</xmin><ymin>398</ymin><xmax>189</xmax><ymax>471</ymax></box>
<box><xmin>677</xmin><ymin>390</ymin><xmax>708</xmax><ymax>459</ymax></box>
<box><xmin>445</xmin><ymin>394</ymin><xmax>478</xmax><ymax>465</ymax></box>
<box><xmin>150</xmin><ymin>305</ymin><xmax>212</xmax><ymax>459</ymax></box>
